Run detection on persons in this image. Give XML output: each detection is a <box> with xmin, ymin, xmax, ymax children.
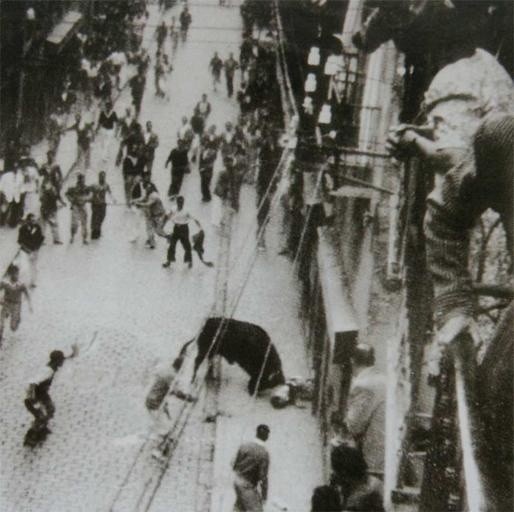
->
<box><xmin>145</xmin><ymin>358</ymin><xmax>201</xmax><ymax>437</ymax></box>
<box><xmin>312</xmin><ymin>342</ymin><xmax>388</xmax><ymax>512</ymax></box>
<box><xmin>232</xmin><ymin>424</ymin><xmax>270</xmax><ymax>512</ymax></box>
<box><xmin>2</xmin><ymin>263</ymin><xmax>31</xmax><ymax>332</ymax></box>
<box><xmin>270</xmin><ymin>376</ymin><xmax>314</xmax><ymax>408</ymax></box>
<box><xmin>2</xmin><ymin>2</ymin><xmax>276</xmax><ymax>269</ymax></box>
<box><xmin>23</xmin><ymin>349</ymin><xmax>64</xmax><ymax>445</ymax></box>
<box><xmin>352</xmin><ymin>3</ymin><xmax>514</xmax><ymax>354</ymax></box>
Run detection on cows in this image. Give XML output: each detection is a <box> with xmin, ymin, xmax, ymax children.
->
<box><xmin>191</xmin><ymin>317</ymin><xmax>289</xmax><ymax>398</ymax></box>
